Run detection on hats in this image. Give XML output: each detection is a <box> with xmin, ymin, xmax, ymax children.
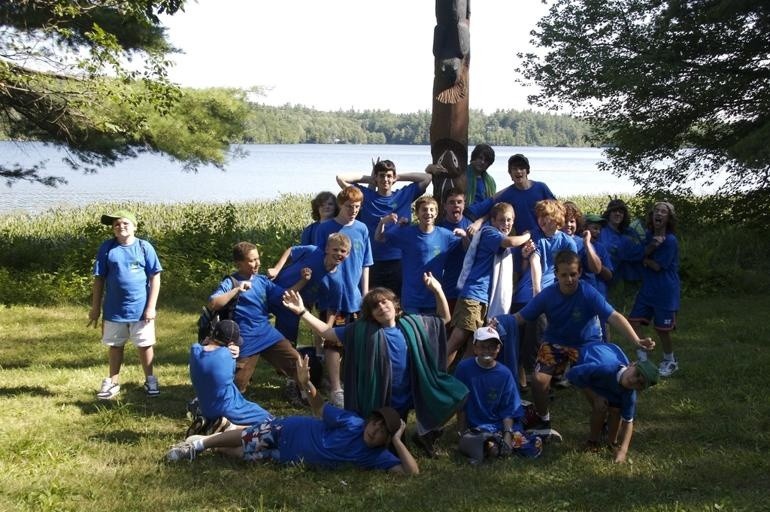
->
<box><xmin>636</xmin><ymin>359</ymin><xmax>660</xmax><ymax>391</ymax></box>
<box><xmin>371</xmin><ymin>406</ymin><xmax>401</xmax><ymax>432</ymax></box>
<box><xmin>213</xmin><ymin>319</ymin><xmax>244</xmax><ymax>347</ymax></box>
<box><xmin>584</xmin><ymin>213</ymin><xmax>609</xmax><ymax>228</ymax></box>
<box><xmin>100</xmin><ymin>210</ymin><xmax>138</xmax><ymax>228</ymax></box>
<box><xmin>472</xmin><ymin>326</ymin><xmax>503</xmax><ymax>346</ymax></box>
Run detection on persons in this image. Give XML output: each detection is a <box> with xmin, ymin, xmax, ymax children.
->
<box><xmin>265</xmin><ymin>143</ymin><xmax>685</xmax><ymax>467</ymax></box>
<box><xmin>186</xmin><ymin>318</ymin><xmax>275</xmax><ymax>438</ymax></box>
<box><xmin>207</xmin><ymin>241</ymin><xmax>327</xmax><ymax>408</ymax></box>
<box><xmin>159</xmin><ymin>354</ymin><xmax>421</xmax><ymax>477</ymax></box>
<box><xmin>85</xmin><ymin>208</ymin><xmax>164</xmax><ymax>399</ymax></box>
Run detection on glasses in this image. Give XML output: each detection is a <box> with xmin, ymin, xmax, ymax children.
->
<box><xmin>475</xmin><ymin>344</ymin><xmax>500</xmax><ymax>351</ymax></box>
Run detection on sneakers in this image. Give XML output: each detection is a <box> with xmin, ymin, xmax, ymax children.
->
<box><xmin>96</xmin><ymin>377</ymin><xmax>120</xmax><ymax>400</ymax></box>
<box><xmin>143</xmin><ymin>375</ymin><xmax>160</xmax><ymax>398</ymax></box>
<box><xmin>205</xmin><ymin>415</ymin><xmax>232</xmax><ymax>436</ymax></box>
<box><xmin>184</xmin><ymin>431</ymin><xmax>223</xmax><ymax>445</ymax></box>
<box><xmin>185</xmin><ymin>415</ymin><xmax>210</xmax><ymax>439</ymax></box>
<box><xmin>556</xmin><ymin>378</ymin><xmax>573</xmax><ymax>387</ymax></box>
<box><xmin>526</xmin><ymin>428</ymin><xmax>563</xmax><ymax>443</ymax></box>
<box><xmin>523</xmin><ymin>417</ymin><xmax>552</xmax><ymax>430</ymax></box>
<box><xmin>412</xmin><ymin>429</ymin><xmax>438</xmax><ymax>459</ymax></box>
<box><xmin>170</xmin><ymin>442</ymin><xmax>198</xmax><ymax>463</ymax></box>
<box><xmin>658</xmin><ymin>359</ymin><xmax>679</xmax><ymax>377</ymax></box>
<box><xmin>330</xmin><ymin>388</ymin><xmax>345</xmax><ymax>407</ymax></box>
